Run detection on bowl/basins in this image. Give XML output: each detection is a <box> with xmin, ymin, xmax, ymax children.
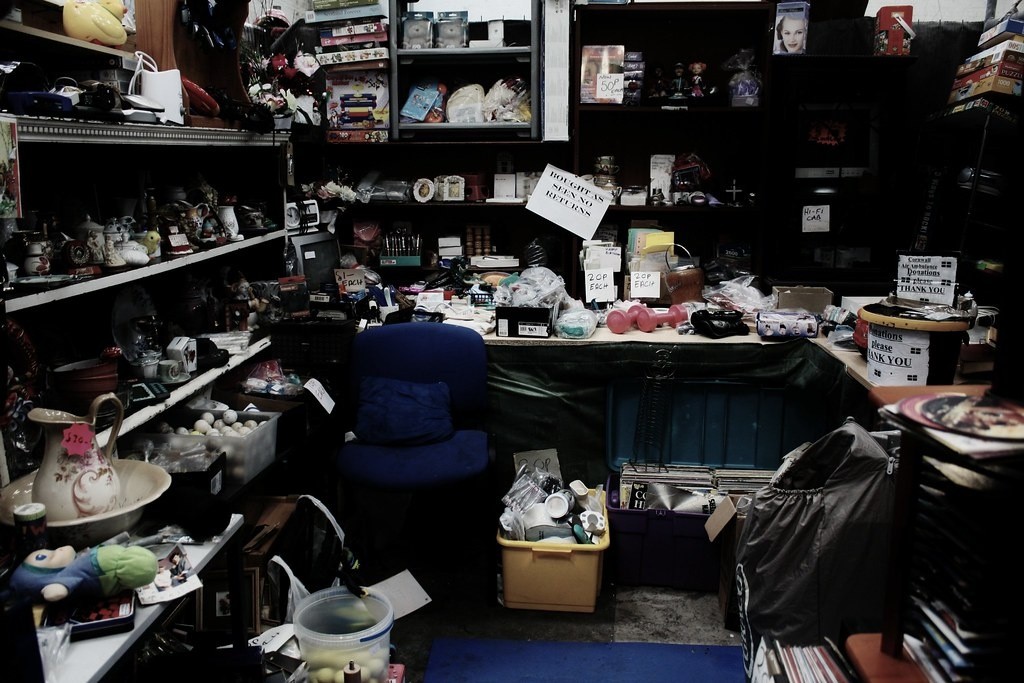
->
<box><xmin>0</xmin><ymin>459</ymin><xmax>171</xmax><ymax>548</ymax></box>
<box><xmin>53</xmin><ymin>357</ymin><xmax>119</xmax><ymax>400</ymax></box>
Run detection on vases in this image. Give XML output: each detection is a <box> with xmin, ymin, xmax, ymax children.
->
<box><xmin>273</xmin><ymin>114</ymin><xmax>294</xmax><ymax>130</ymax></box>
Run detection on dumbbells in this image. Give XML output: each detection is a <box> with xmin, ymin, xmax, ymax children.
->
<box><xmin>605</xmin><ymin>304</ymin><xmax>689</xmax><ymax>334</ymax></box>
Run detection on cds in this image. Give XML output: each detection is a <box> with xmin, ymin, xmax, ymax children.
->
<box><xmin>898</xmin><ymin>392</ymin><xmax>1024</xmax><ymax>442</ymax></box>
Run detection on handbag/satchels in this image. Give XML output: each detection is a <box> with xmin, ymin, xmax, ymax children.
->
<box><xmin>735</xmin><ymin>416</ymin><xmax>898</xmax><ymax>683</ymax></box>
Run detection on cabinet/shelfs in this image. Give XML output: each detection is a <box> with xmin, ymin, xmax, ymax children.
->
<box><xmin>896</xmin><ymin>92</ymin><xmax>1024</xmax><ymax>292</ymax></box>
<box><xmin>845</xmin><ymin>383</ymin><xmax>1024</xmax><ymax>683</ymax></box>
<box><xmin>0</xmin><ymin>0</ymin><xmax>781</xmax><ymax>683</ymax></box>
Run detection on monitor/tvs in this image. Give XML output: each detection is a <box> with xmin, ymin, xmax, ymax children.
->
<box><xmin>286</xmin><ymin>232</ymin><xmax>343</xmax><ymax>294</ymax></box>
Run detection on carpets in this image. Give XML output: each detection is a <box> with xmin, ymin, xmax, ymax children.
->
<box><xmin>423</xmin><ymin>634</ymin><xmax>748</xmax><ymax>683</ymax></box>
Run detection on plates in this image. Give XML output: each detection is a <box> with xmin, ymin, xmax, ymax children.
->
<box><xmin>157</xmin><ymin>373</ymin><xmax>191</xmax><ymax>384</ymax></box>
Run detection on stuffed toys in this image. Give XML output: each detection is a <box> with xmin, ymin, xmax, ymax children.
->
<box><xmin>11</xmin><ymin>545</ymin><xmax>158</xmax><ymax>603</ymax></box>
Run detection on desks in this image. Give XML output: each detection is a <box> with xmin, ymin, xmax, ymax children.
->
<box><xmin>485</xmin><ymin>318</ymin><xmax>994</xmax><ymax>387</ymax></box>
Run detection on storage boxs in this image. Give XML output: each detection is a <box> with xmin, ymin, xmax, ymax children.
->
<box><xmin>496</xmin><ymin>487</ymin><xmax>610</xmax><ymax>612</ymax></box>
<box><xmin>403</xmin><ymin>11</ymin><xmax>434</xmax><ymax>49</ymax></box>
<box><xmin>469</xmin><ymin>40</ymin><xmax>507</xmax><ymax>48</ymax></box>
<box><xmin>315</xmin><ymin>48</ymin><xmax>390</xmax><ymax>65</ymax></box>
<box><xmin>772</xmin><ymin>285</ymin><xmax>835</xmax><ymax>313</ymax></box>
<box><xmin>604</xmin><ymin>370</ymin><xmax>797</xmax><ymax>592</ymax></box>
<box><xmin>312</xmin><ymin>0</ymin><xmax>379</xmax><ymax>12</ymax></box>
<box><xmin>322</xmin><ymin>68</ymin><xmax>390</xmax><ymax>129</ymax></box>
<box><xmin>438</xmin><ymin>245</ymin><xmax>465</xmax><ymax>256</ymax></box>
<box><xmin>947</xmin><ymin>18</ymin><xmax>1024</xmax><ymax>103</ymax></box>
<box><xmin>495</xmin><ymin>299</ymin><xmax>563</xmax><ymax>338</ymax></box>
<box><xmin>620</xmin><ymin>190</ymin><xmax>648</xmax><ymax>205</ymax></box>
<box><xmin>772</xmin><ymin>2</ymin><xmax>810</xmax><ymax>55</ymax></box>
<box><xmin>842</xmin><ymin>296</ymin><xmax>888</xmax><ymax>332</ymax></box>
<box><xmin>326</xmin><ymin>131</ymin><xmax>389</xmax><ymax>142</ymax></box>
<box><xmin>127</xmin><ymin>408</ymin><xmax>281</xmax><ymax>484</ymax></box>
<box><xmin>214</xmin><ymin>390</ymin><xmax>307</xmax><ymax>460</ymax></box>
<box><xmin>305</xmin><ymin>5</ymin><xmax>388</xmax><ymax>24</ymax></box>
<box><xmin>895</xmin><ymin>249</ymin><xmax>973</xmax><ymax>310</ymax></box>
<box><xmin>146</xmin><ymin>449</ymin><xmax>228</xmax><ymax>515</ymax></box>
<box><xmin>438</xmin><ymin>236</ymin><xmax>461</xmax><ymax>247</ymax></box>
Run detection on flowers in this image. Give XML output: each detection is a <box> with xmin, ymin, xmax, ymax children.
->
<box><xmin>239</xmin><ymin>0</ymin><xmax>298</xmax><ymax>120</ymax></box>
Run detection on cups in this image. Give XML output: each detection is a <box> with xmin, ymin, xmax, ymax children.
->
<box><xmin>159</xmin><ymin>360</ymin><xmax>184</xmax><ymax>381</ymax></box>
<box><xmin>131</xmin><ymin>353</ymin><xmax>159</xmax><ymax>379</ymax></box>
<box><xmin>545</xmin><ymin>489</ymin><xmax>576</xmax><ymax>518</ymax></box>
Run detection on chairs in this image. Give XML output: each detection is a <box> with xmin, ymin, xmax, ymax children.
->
<box><xmin>329</xmin><ymin>322</ymin><xmax>491</xmax><ymax>633</ymax></box>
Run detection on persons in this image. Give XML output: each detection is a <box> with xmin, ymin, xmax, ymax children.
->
<box><xmin>776</xmin><ymin>16</ymin><xmax>805</xmax><ymax>53</ymax></box>
<box><xmin>647</xmin><ymin>63</ymin><xmax>707</xmax><ymax>98</ymax></box>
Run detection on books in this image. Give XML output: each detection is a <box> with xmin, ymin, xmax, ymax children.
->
<box><xmin>902</xmin><ymin>454</ymin><xmax>1024</xmax><ymax>683</ymax></box>
<box><xmin>628</xmin><ymin>228</ymin><xmax>674</xmax><ymax>257</ymax></box>
<box><xmin>618</xmin><ymin>464</ymin><xmax>777</xmax><ymax>510</ymax></box>
<box><xmin>752</xmin><ymin>636</ymin><xmax>855</xmax><ymax>683</ymax></box>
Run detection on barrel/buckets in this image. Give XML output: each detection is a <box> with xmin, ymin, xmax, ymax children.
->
<box><xmin>293</xmin><ymin>586</ymin><xmax>394</xmax><ymax>682</ymax></box>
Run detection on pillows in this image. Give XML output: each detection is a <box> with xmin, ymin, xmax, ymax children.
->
<box><xmin>354</xmin><ymin>374</ymin><xmax>458</xmax><ymax>446</ymax></box>
